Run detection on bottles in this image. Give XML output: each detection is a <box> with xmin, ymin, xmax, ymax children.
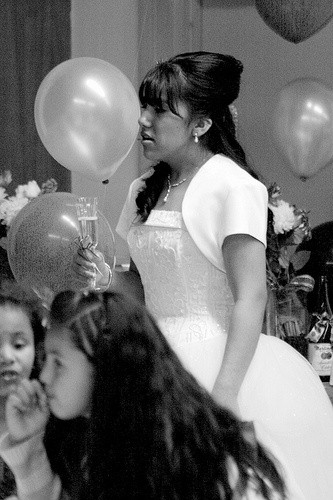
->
<box><xmin>306</xmin><ymin>276</ymin><xmax>333</xmax><ymax>382</ymax></box>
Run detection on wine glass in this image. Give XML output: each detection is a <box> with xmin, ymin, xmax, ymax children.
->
<box><xmin>74</xmin><ymin>194</ymin><xmax>101</xmax><ymax>291</ymax></box>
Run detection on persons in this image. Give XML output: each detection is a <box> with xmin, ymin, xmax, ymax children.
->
<box><xmin>70</xmin><ymin>47</ymin><xmax>332</xmax><ymax>500</ymax></box>
<box><xmin>0</xmin><ymin>281</ymin><xmax>287</xmax><ymax>500</ymax></box>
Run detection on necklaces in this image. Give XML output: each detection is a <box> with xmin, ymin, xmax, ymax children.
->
<box><xmin>164</xmin><ymin>172</ymin><xmax>186</xmax><ymax>203</ymax></box>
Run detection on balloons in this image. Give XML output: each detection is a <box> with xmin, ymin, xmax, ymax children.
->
<box><xmin>35</xmin><ymin>57</ymin><xmax>142</xmax><ymax>186</ymax></box>
<box><xmin>4</xmin><ymin>192</ymin><xmax>114</xmax><ymax>306</ymax></box>
<box><xmin>256</xmin><ymin>0</ymin><xmax>332</xmax><ymax>44</ymax></box>
<box><xmin>270</xmin><ymin>78</ymin><xmax>332</xmax><ymax>179</ymax></box>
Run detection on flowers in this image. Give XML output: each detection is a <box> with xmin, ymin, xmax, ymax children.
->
<box><xmin>266</xmin><ymin>177</ymin><xmax>310</xmax><ymax>288</ymax></box>
<box><xmin>0</xmin><ymin>179</ymin><xmax>41</xmax><ymax>224</ymax></box>
